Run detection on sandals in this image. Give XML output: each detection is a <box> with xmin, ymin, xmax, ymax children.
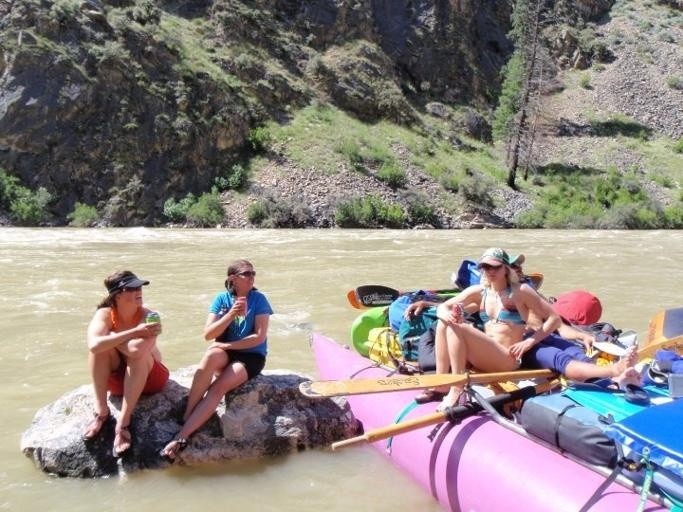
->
<box><xmin>112</xmin><ymin>414</ymin><xmax>133</xmax><ymax>458</ymax></box>
<box><xmin>82</xmin><ymin>406</ymin><xmax>113</xmax><ymax>442</ymax></box>
<box><xmin>436</xmin><ymin>387</ymin><xmax>465</xmax><ymax>412</ymax></box>
<box><xmin>159</xmin><ymin>420</ymin><xmax>191</xmax><ymax>465</ymax></box>
<box><xmin>415</xmin><ymin>389</ymin><xmax>449</xmax><ymax>404</ymax></box>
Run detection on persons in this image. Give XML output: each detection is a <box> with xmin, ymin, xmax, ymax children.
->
<box><xmin>159</xmin><ymin>259</ymin><xmax>274</xmax><ymax>465</ymax></box>
<box><xmin>81</xmin><ymin>270</ymin><xmax>169</xmax><ymax>458</ymax></box>
<box><xmin>404</xmin><ymin>253</ymin><xmax>643</xmax><ymax>388</ymax></box>
<box><xmin>415</xmin><ymin>247</ymin><xmax>562</xmax><ymax>409</ymax></box>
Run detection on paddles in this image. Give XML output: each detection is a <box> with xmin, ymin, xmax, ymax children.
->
<box><xmin>331</xmin><ymin>336</ymin><xmax>682</xmax><ymax>454</ymax></box>
<box><xmin>300</xmin><ymin>369</ymin><xmax>560</xmax><ymax>398</ymax></box>
<box><xmin>348</xmin><ymin>274</ymin><xmax>542</xmax><ymax>308</ymax></box>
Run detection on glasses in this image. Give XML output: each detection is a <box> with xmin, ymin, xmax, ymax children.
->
<box><xmin>482</xmin><ymin>264</ymin><xmax>503</xmax><ymax>271</ymax></box>
<box><xmin>236</xmin><ymin>270</ymin><xmax>256</xmax><ymax>278</ymax></box>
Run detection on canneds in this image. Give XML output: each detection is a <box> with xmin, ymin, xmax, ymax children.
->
<box><xmin>452</xmin><ymin>303</ymin><xmax>464</xmax><ymax>323</ymax></box>
<box><xmin>145</xmin><ymin>311</ymin><xmax>161</xmax><ymax>334</ymax></box>
<box><xmin>238</xmin><ymin>296</ymin><xmax>247</xmax><ymax>315</ymax></box>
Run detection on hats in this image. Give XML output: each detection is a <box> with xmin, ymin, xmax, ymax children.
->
<box><xmin>108</xmin><ymin>274</ymin><xmax>151</xmax><ymax>293</ymax></box>
<box><xmin>478</xmin><ymin>247</ymin><xmax>510</xmax><ymax>269</ymax></box>
<box><xmin>507</xmin><ymin>253</ymin><xmax>526</xmax><ymax>266</ymax></box>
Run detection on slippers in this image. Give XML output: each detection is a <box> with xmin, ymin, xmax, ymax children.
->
<box><xmin>624</xmin><ymin>383</ymin><xmax>650</xmax><ymax>405</ymax></box>
<box><xmin>567</xmin><ymin>376</ymin><xmax>625</xmax><ymax>393</ymax></box>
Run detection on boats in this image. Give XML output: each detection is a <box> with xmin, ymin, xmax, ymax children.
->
<box><xmin>305</xmin><ymin>286</ymin><xmax>683</xmax><ymax>512</ymax></box>
